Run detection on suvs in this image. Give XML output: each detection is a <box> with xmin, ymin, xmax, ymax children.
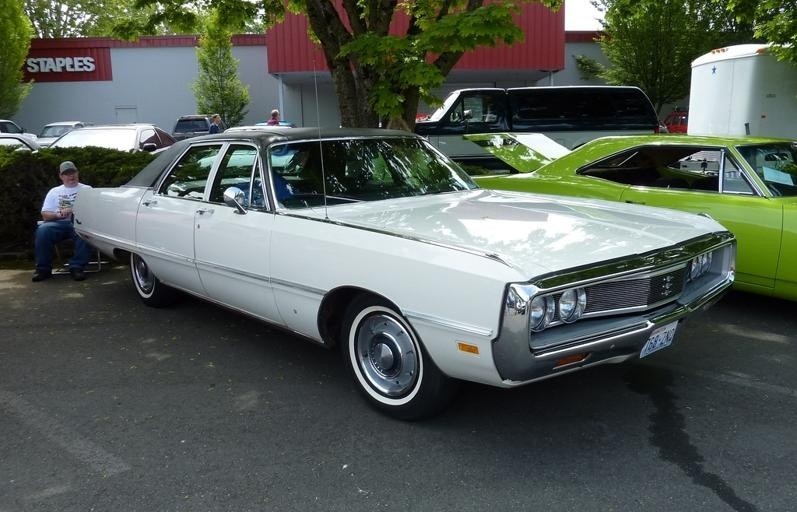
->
<box><xmin>171</xmin><ymin>114</ymin><xmax>228</xmax><ymax>139</ymax></box>
<box><xmin>656</xmin><ymin>111</ymin><xmax>687</xmax><ymax>133</ymax></box>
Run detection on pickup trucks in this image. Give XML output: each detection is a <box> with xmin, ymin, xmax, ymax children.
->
<box><xmin>38</xmin><ymin>120</ymin><xmax>91</xmax><ymax>150</ymax></box>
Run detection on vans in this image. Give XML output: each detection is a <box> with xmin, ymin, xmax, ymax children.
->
<box><xmin>412</xmin><ymin>84</ymin><xmax>659</xmax><ymax>156</ymax></box>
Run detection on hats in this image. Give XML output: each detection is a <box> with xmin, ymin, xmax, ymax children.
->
<box><xmin>60</xmin><ymin>161</ymin><xmax>77</xmax><ymax>175</ymax></box>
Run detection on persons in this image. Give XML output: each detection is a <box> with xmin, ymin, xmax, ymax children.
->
<box><xmin>267</xmin><ymin>110</ymin><xmax>280</xmax><ymax>126</ymax></box>
<box><xmin>209</xmin><ymin>113</ymin><xmax>221</xmax><ymax>134</ymax></box>
<box><xmin>31</xmin><ymin>161</ymin><xmax>94</xmax><ymax>281</ymax></box>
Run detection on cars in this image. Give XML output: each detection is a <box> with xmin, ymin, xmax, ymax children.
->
<box><xmin>255</xmin><ymin>121</ymin><xmax>295</xmax><ymax>130</ymax></box>
<box><xmin>67</xmin><ymin>125</ymin><xmax>736</xmax><ymax>421</ymax></box>
<box><xmin>0</xmin><ymin>119</ymin><xmax>33</xmax><ymax>133</ymax></box>
<box><xmin>447</xmin><ymin>124</ymin><xmax>797</xmax><ymax>304</ymax></box>
<box><xmin>0</xmin><ymin>133</ymin><xmax>41</xmax><ymax>156</ymax></box>
<box><xmin>38</xmin><ymin>125</ymin><xmax>178</xmax><ymax>157</ymax></box>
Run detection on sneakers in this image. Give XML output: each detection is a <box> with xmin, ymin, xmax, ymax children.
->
<box><xmin>71</xmin><ymin>268</ymin><xmax>84</xmax><ymax>280</ymax></box>
<box><xmin>32</xmin><ymin>272</ymin><xmax>49</xmax><ymax>282</ymax></box>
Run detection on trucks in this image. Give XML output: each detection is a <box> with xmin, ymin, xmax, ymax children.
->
<box><xmin>686</xmin><ymin>43</ymin><xmax>797</xmax><ymax>139</ymax></box>
